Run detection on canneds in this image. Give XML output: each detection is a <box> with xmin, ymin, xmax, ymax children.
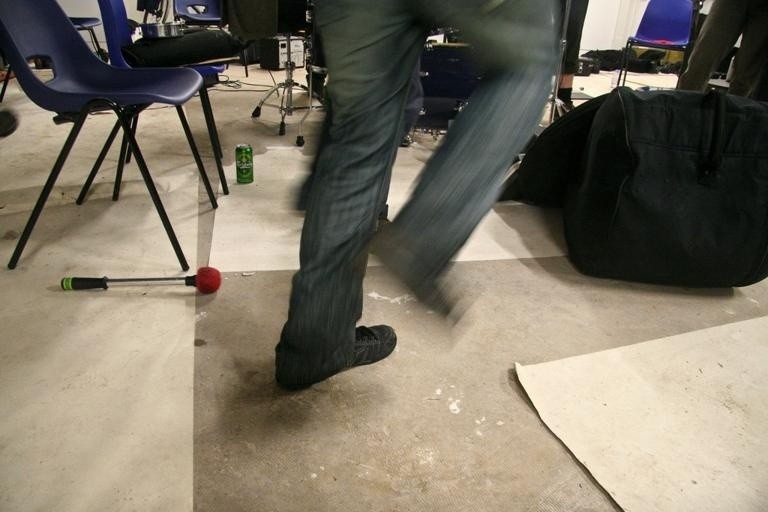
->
<box><xmin>235</xmin><ymin>143</ymin><xmax>254</xmax><ymax>184</ymax></box>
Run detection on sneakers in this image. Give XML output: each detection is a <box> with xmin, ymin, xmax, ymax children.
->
<box><xmin>271</xmin><ymin>323</ymin><xmax>398</xmax><ymax>390</ymax></box>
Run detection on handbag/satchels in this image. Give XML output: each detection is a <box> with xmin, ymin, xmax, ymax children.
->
<box><xmin>497</xmin><ymin>80</ymin><xmax>765</xmax><ymax>295</ymax></box>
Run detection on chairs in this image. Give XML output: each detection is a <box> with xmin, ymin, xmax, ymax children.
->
<box><xmin>616</xmin><ymin>1</ymin><xmax>695</xmax><ymax>91</ymax></box>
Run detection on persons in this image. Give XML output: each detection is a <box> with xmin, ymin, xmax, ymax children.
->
<box><xmin>272</xmin><ymin>1</ymin><xmax>573</xmax><ymax>396</ymax></box>
<box><xmin>553</xmin><ymin>0</ymin><xmax>590</xmax><ymax>124</ymax></box>
<box><xmin>676</xmin><ymin>0</ymin><xmax>767</xmax><ymax>101</ymax></box>
